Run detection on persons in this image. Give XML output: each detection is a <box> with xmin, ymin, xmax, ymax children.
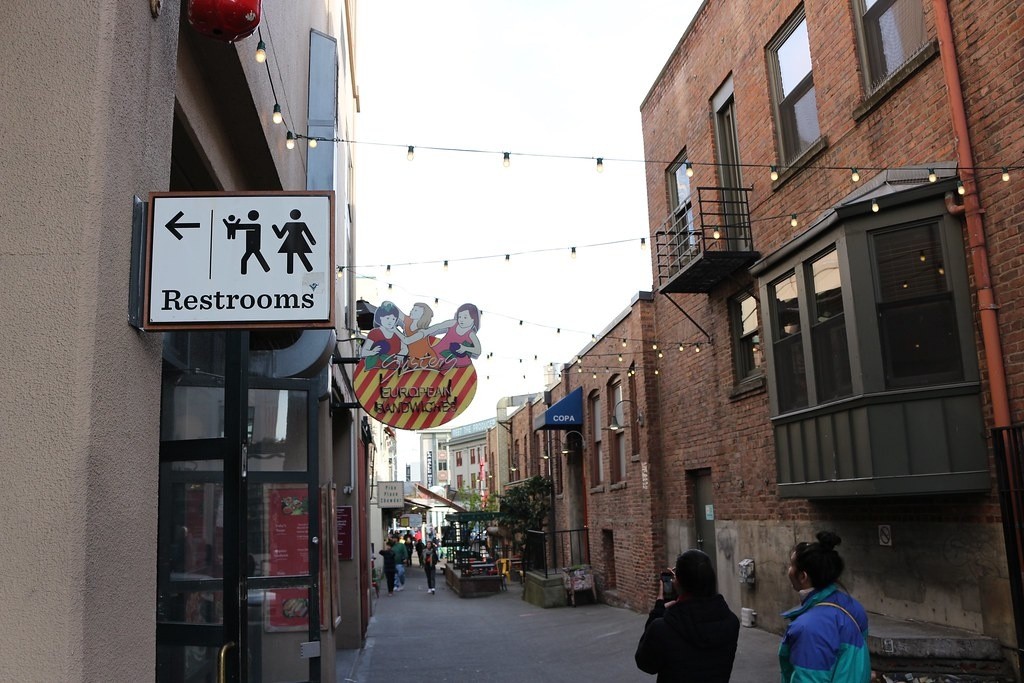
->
<box><xmin>378</xmin><ymin>530</ymin><xmax>441</xmax><ymax>595</ymax></box>
<box><xmin>635</xmin><ymin>549</ymin><xmax>741</xmax><ymax>683</ymax></box>
<box><xmin>778</xmin><ymin>530</ymin><xmax>874</xmax><ymax>683</ymax></box>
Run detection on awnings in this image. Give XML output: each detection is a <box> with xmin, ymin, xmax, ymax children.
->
<box><xmin>402</xmin><ymin>498</ymin><xmax>432</xmax><ymax>513</ymax></box>
<box><xmin>533</xmin><ymin>384</ymin><xmax>584</xmax><ymax>431</ymax></box>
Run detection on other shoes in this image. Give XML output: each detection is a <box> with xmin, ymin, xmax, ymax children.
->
<box><xmin>432</xmin><ymin>588</ymin><xmax>435</xmax><ymax>595</ymax></box>
<box><xmin>387</xmin><ymin>591</ymin><xmax>393</xmax><ymax>596</ymax></box>
<box><xmin>427</xmin><ymin>589</ymin><xmax>432</xmax><ymax>593</ymax></box>
<box><xmin>394</xmin><ymin>586</ymin><xmax>398</xmax><ymax>591</ymax></box>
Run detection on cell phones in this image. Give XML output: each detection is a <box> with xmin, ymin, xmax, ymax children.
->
<box><xmin>661</xmin><ymin>572</ymin><xmax>676</xmax><ymax>602</ymax></box>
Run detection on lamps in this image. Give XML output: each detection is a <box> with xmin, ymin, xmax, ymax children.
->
<box><xmin>474</xmin><ymin>431</ymin><xmax>587</xmax><ymax>483</ymax></box>
<box><xmin>601</xmin><ymin>399</ymin><xmax>644</xmax><ymax>430</ymax></box>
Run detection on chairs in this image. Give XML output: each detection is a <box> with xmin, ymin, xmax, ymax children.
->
<box><xmin>456</xmin><ymin>557</ymin><xmax>533</xmax><ymax>576</ymax></box>
<box><xmin>495</xmin><ymin>559</ymin><xmax>512</xmax><ymax>585</ymax></box>
